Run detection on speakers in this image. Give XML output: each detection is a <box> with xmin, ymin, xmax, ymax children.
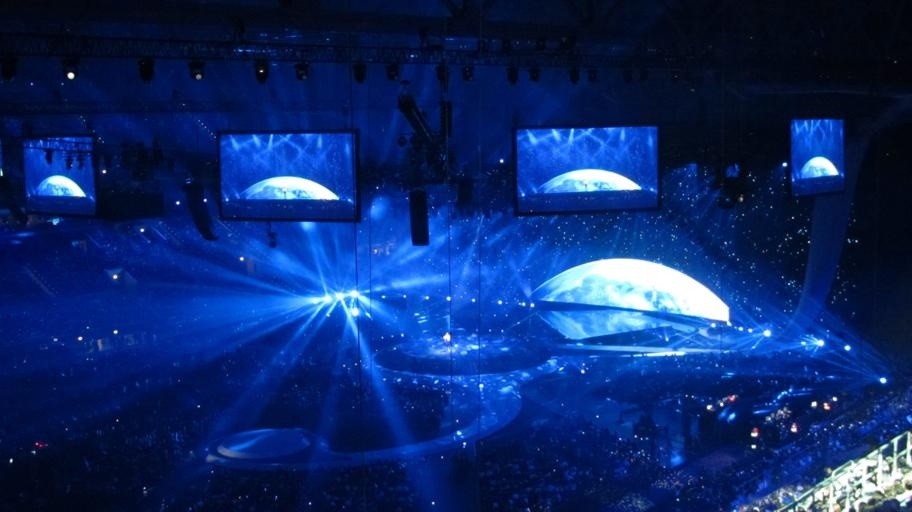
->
<box><xmin>184</xmin><ymin>181</ymin><xmax>219</xmax><ymax>242</ymax></box>
<box><xmin>408</xmin><ymin>190</ymin><xmax>432</xmax><ymax>247</ymax></box>
